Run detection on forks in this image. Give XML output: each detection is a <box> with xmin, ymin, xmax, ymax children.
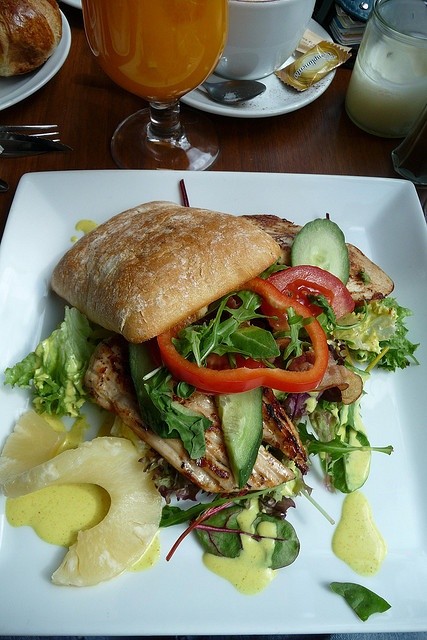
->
<box><xmin>1</xmin><ymin>124</ymin><xmax>60</xmax><ymax>156</ymax></box>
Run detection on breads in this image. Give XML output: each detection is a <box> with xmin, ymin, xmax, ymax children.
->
<box><xmin>49</xmin><ymin>197</ymin><xmax>283</xmax><ymax>345</ymax></box>
<box><xmin>0</xmin><ymin>0</ymin><xmax>62</xmax><ymax>79</ymax></box>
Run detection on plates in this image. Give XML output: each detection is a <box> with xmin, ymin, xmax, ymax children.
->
<box><xmin>0</xmin><ymin>7</ymin><xmax>72</xmax><ymax>110</ymax></box>
<box><xmin>1</xmin><ymin>168</ymin><xmax>426</xmax><ymax>636</ymax></box>
<box><xmin>182</xmin><ymin>17</ymin><xmax>337</xmax><ymax>118</ymax></box>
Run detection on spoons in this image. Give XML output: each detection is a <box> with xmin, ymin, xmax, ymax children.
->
<box><xmin>197</xmin><ymin>79</ymin><xmax>266</xmax><ymax>102</ymax></box>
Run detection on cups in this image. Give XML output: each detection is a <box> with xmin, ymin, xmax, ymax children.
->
<box><xmin>213</xmin><ymin>0</ymin><xmax>316</xmax><ymax>82</ymax></box>
<box><xmin>392</xmin><ymin>110</ymin><xmax>427</xmax><ymax>179</ymax></box>
<box><xmin>346</xmin><ymin>0</ymin><xmax>426</xmax><ymax>138</ymax></box>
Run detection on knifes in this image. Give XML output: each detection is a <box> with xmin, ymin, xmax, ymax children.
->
<box><xmin>0</xmin><ymin>133</ymin><xmax>73</xmax><ymax>157</ymax></box>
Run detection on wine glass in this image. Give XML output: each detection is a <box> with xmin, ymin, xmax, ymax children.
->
<box><xmin>80</xmin><ymin>0</ymin><xmax>228</xmax><ymax>170</ymax></box>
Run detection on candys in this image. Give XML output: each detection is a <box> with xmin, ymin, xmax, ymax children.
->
<box><xmin>296</xmin><ymin>28</ymin><xmax>351</xmax><ymax>55</ymax></box>
<box><xmin>274</xmin><ymin>40</ymin><xmax>351</xmax><ymax>91</ymax></box>
